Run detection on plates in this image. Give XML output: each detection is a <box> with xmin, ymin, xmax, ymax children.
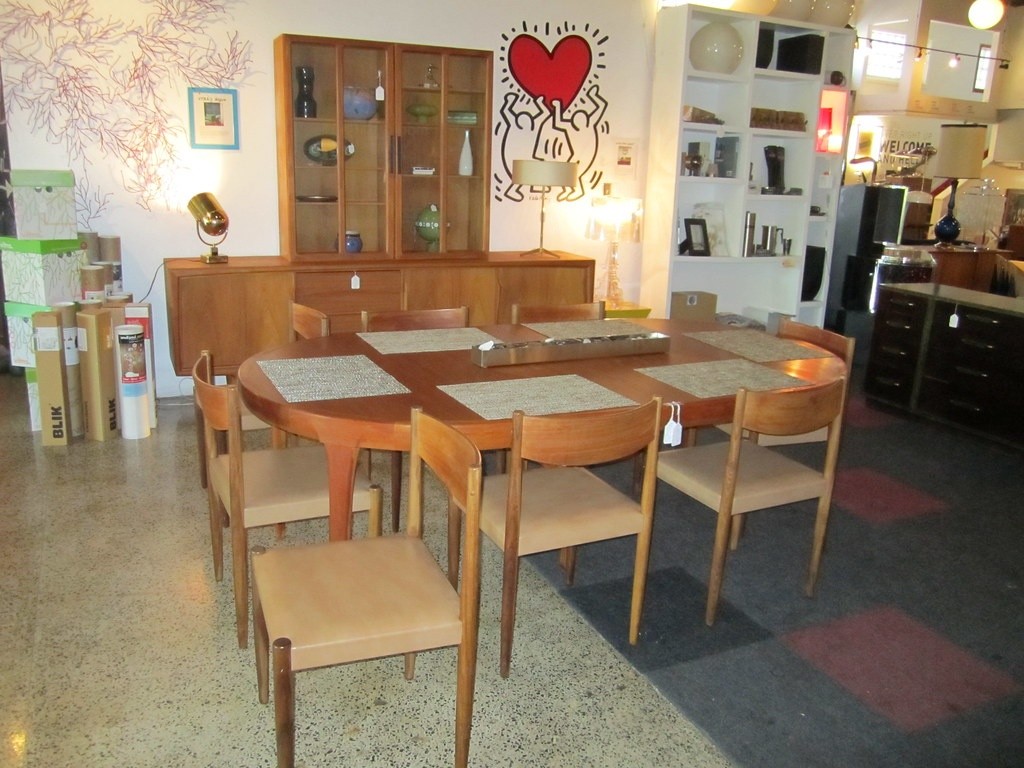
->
<box><xmin>295</xmin><ymin>196</ymin><xmax>340</xmax><ymax>202</ymax></box>
<box><xmin>303</xmin><ymin>134</ymin><xmax>355</xmax><ymax>164</ymax></box>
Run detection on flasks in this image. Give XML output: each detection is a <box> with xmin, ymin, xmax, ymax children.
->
<box><xmin>743</xmin><ymin>211</ymin><xmax>756</xmax><ymax>257</ymax></box>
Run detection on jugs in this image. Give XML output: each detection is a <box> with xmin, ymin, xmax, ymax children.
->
<box><xmin>762</xmin><ymin>225</ymin><xmax>783</xmax><ymax>251</ymax></box>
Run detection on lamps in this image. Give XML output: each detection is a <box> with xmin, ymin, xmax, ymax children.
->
<box><xmin>848</xmin><ymin>147</ymin><xmax>931</xmax><ymax>182</ymax></box>
<box><xmin>867</xmin><ymin>39</ymin><xmax>1009</xmax><ymax>69</ymax></box>
<box><xmin>187</xmin><ymin>192</ymin><xmax>229</xmax><ymax>265</ymax></box>
<box><xmin>591</xmin><ymin>194</ymin><xmax>642</xmax><ymax>308</ymax></box>
<box><xmin>513</xmin><ymin>160</ymin><xmax>577</xmax><ymax>258</ymax></box>
<box><xmin>930</xmin><ymin>124</ymin><xmax>988</xmax><ymax>249</ymax></box>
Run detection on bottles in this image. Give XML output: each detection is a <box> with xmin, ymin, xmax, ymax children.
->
<box><xmin>336</xmin><ymin>232</ymin><xmax>363</xmax><ymax>253</ymax></box>
<box><xmin>458</xmin><ymin>130</ymin><xmax>473</xmax><ymax>175</ymax></box>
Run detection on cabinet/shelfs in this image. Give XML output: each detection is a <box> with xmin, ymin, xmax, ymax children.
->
<box><xmin>275</xmin><ymin>34</ymin><xmax>494</xmax><ymax>263</ymax></box>
<box><xmin>163</xmin><ymin>249</ymin><xmax>596</xmax><ymax>386</ymax></box>
<box><xmin>640</xmin><ymin>4</ymin><xmax>857</xmax><ymax>329</ymax></box>
<box><xmin>864</xmin><ymin>245</ymin><xmax>1024</xmax><ymax>453</ymax></box>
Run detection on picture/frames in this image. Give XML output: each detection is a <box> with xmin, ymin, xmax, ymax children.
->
<box><xmin>683</xmin><ymin>217</ymin><xmax>710</xmax><ymax>257</ymax></box>
<box><xmin>188</xmin><ymin>87</ymin><xmax>240</xmax><ymax>149</ymax></box>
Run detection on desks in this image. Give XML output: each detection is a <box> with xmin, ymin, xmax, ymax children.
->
<box><xmin>604</xmin><ymin>300</ymin><xmax>651</xmax><ymax>318</ymax></box>
<box><xmin>236</xmin><ymin>320</ymin><xmax>847</xmax><ymax>565</ymax></box>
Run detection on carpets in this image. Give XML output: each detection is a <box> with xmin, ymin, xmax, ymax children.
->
<box><xmin>482</xmin><ymin>376</ymin><xmax>1024</xmax><ymax>768</ymax></box>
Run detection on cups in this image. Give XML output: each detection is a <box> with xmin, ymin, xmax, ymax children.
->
<box><xmin>783</xmin><ymin>239</ymin><xmax>791</xmax><ymax>255</ymax></box>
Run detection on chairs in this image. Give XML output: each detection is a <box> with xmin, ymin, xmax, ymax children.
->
<box><xmin>190</xmin><ymin>303</ymin><xmax>856</xmax><ymax>768</ymax></box>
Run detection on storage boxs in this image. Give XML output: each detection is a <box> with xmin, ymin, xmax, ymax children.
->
<box><xmin>670</xmin><ymin>291</ymin><xmax>718</xmax><ymax>322</ymax></box>
<box><xmin>0</xmin><ymin>169</ymin><xmax>157</xmax><ymax>448</ymax></box>
<box><xmin>887</xmin><ymin>174</ymin><xmax>933</xmax><ymax>241</ymax></box>
